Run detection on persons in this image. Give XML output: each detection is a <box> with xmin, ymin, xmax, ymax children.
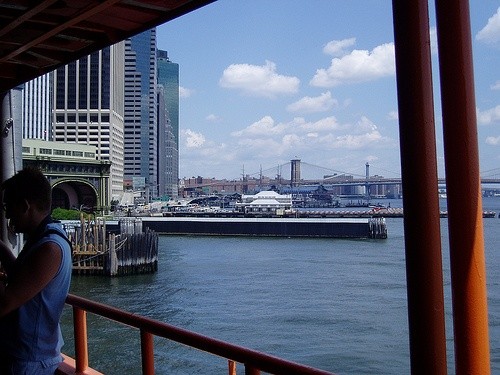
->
<box><xmin>0</xmin><ymin>166</ymin><xmax>74</xmax><ymax>375</ymax></box>
